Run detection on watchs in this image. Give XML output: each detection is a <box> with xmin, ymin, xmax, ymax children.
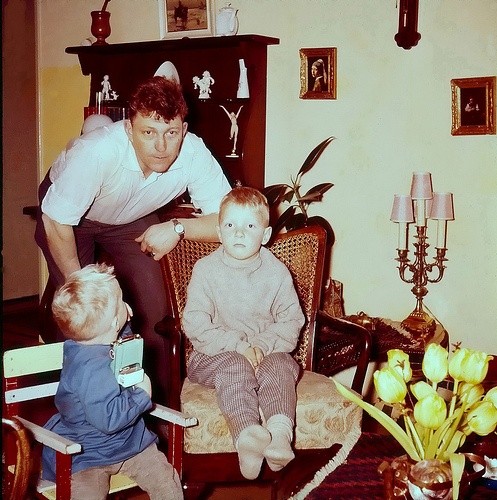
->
<box><xmin>170</xmin><ymin>218</ymin><xmax>186</xmax><ymax>241</ymax></box>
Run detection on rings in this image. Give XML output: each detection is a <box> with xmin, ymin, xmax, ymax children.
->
<box><xmin>151</xmin><ymin>251</ymin><xmax>157</xmax><ymax>257</ymax></box>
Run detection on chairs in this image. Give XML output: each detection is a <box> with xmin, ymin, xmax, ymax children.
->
<box><xmin>156</xmin><ymin>223</ymin><xmax>364</xmax><ymax>500</ymax></box>
<box><xmin>0</xmin><ymin>342</ymin><xmax>198</xmax><ymax>500</ymax></box>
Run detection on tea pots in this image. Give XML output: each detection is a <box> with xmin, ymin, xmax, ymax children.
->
<box><xmin>215</xmin><ymin>2</ymin><xmax>238</xmax><ymax>35</ymax></box>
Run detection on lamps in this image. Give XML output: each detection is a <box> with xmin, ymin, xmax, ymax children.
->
<box><xmin>391</xmin><ymin>172</ymin><xmax>455</xmax><ymax>331</ymax></box>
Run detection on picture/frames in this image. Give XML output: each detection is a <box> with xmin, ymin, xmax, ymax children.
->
<box><xmin>450</xmin><ymin>76</ymin><xmax>497</xmax><ymax>137</ymax></box>
<box><xmin>299</xmin><ymin>47</ymin><xmax>337</xmax><ymax>100</ymax></box>
<box><xmin>157</xmin><ymin>1</ymin><xmax>216</xmax><ymax>40</ymax></box>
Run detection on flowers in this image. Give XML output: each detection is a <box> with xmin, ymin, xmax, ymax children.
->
<box><xmin>329</xmin><ymin>343</ymin><xmax>497</xmax><ymax>500</ymax></box>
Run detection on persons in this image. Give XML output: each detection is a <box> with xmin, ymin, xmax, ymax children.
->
<box><xmin>192</xmin><ymin>69</ymin><xmax>218</xmax><ymax>101</ymax></box>
<box><xmin>33</xmin><ymin>77</ymin><xmax>233</xmax><ymax>403</ymax></box>
<box><xmin>182</xmin><ymin>186</ymin><xmax>307</xmax><ymax>480</ymax></box>
<box><xmin>42</xmin><ymin>263</ymin><xmax>183</xmax><ymax>500</ymax></box>
<box><xmin>459</xmin><ymin>88</ymin><xmax>485</xmax><ymax>123</ymax></box>
<box><xmin>306</xmin><ymin>55</ymin><xmax>330</xmax><ymax>93</ymax></box>
<box><xmin>94</xmin><ymin>72</ymin><xmax>117</xmax><ymax>103</ymax></box>
<box><xmin>218</xmin><ymin>105</ymin><xmax>244</xmax><ymax>154</ymax></box>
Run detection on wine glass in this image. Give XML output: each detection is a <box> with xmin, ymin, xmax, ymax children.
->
<box><xmin>90</xmin><ymin>10</ymin><xmax>111</xmax><ymax>46</ymax></box>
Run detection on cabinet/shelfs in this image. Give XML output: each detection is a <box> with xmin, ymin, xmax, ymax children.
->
<box><xmin>63</xmin><ymin>34</ymin><xmax>281</xmax><ymax>200</ymax></box>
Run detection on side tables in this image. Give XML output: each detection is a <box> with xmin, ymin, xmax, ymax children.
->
<box><xmin>359</xmin><ymin>319</ymin><xmax>450</xmax><ymax>395</ymax></box>
